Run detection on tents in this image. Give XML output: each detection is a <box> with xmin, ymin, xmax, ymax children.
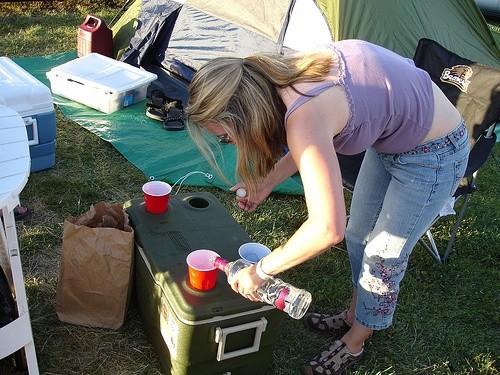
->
<box><xmin>109</xmin><ymin>0</ymin><xmax>500</xmax><ymax>143</ymax></box>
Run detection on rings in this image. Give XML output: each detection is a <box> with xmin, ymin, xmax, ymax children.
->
<box><xmin>245</xmin><ymin>293</ymin><xmax>250</xmax><ymax>295</ymax></box>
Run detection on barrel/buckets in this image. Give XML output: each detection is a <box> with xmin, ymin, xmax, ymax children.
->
<box><xmin>76</xmin><ymin>14</ymin><xmax>113</xmax><ymax>60</ymax></box>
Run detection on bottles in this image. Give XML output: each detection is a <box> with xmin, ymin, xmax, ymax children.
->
<box><xmin>208</xmin><ymin>254</ymin><xmax>312</xmax><ymax>319</ymax></box>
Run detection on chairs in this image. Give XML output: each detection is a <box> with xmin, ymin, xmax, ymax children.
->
<box><xmin>336</xmin><ymin>37</ymin><xmax>500</xmax><ymax>267</ymax></box>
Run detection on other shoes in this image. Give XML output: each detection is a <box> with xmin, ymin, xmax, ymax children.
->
<box><xmin>14</xmin><ymin>206</ymin><xmax>30</xmax><ymax>220</ymax></box>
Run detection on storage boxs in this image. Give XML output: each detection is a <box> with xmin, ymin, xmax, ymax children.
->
<box><xmin>46</xmin><ymin>52</ymin><xmax>158</xmax><ymax>114</ymax></box>
<box><xmin>123</xmin><ymin>191</ymin><xmax>281</xmax><ymax>375</ymax></box>
<box><xmin>0</xmin><ymin>56</ymin><xmax>55</xmax><ymax>173</ymax></box>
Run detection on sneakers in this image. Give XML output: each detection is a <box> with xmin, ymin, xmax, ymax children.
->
<box><xmin>300</xmin><ymin>335</ymin><xmax>365</xmax><ymax>375</ymax></box>
<box><xmin>303</xmin><ymin>307</ymin><xmax>356</xmax><ymax>335</ymax></box>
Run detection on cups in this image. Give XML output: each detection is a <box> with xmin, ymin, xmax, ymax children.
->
<box><xmin>142</xmin><ymin>181</ymin><xmax>172</xmax><ymax>214</ymax></box>
<box><xmin>186</xmin><ymin>249</ymin><xmax>221</xmax><ymax>291</ymax></box>
<box><xmin>238</xmin><ymin>243</ymin><xmax>271</xmax><ymax>264</ymax></box>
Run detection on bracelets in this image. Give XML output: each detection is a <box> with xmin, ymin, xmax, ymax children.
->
<box><xmin>256</xmin><ymin>260</ymin><xmax>273</xmax><ymax>280</ymax></box>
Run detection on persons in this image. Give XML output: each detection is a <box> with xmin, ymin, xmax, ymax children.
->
<box><xmin>0</xmin><ymin>206</ymin><xmax>30</xmax><ymax>220</ymax></box>
<box><xmin>186</xmin><ymin>39</ymin><xmax>470</xmax><ymax>375</ymax></box>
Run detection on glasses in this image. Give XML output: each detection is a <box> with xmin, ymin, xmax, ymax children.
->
<box><xmin>218</xmin><ymin>133</ymin><xmax>231</xmax><ymax>145</ymax></box>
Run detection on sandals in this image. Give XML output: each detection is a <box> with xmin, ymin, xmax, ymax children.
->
<box><xmin>163</xmin><ymin>98</ymin><xmax>186</xmax><ymax>130</ymax></box>
<box><xmin>146</xmin><ymin>88</ymin><xmax>166</xmax><ymax>122</ymax></box>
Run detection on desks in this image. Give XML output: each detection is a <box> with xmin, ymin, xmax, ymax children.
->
<box><xmin>0</xmin><ymin>105</ymin><xmax>40</xmax><ymax>375</ymax></box>
